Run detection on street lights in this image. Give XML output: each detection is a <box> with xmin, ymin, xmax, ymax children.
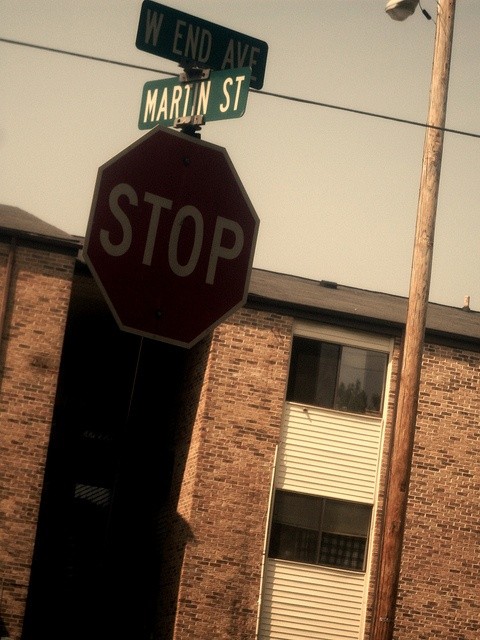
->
<box><xmin>363</xmin><ymin>0</ymin><xmax>456</xmax><ymax>639</ymax></box>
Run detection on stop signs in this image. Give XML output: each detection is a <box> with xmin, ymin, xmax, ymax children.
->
<box><xmin>83</xmin><ymin>123</ymin><xmax>261</xmax><ymax>347</ymax></box>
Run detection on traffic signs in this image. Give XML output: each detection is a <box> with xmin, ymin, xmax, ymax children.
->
<box><xmin>135</xmin><ymin>0</ymin><xmax>271</xmax><ymax>95</ymax></box>
<box><xmin>136</xmin><ymin>66</ymin><xmax>250</xmax><ymax>132</ymax></box>
